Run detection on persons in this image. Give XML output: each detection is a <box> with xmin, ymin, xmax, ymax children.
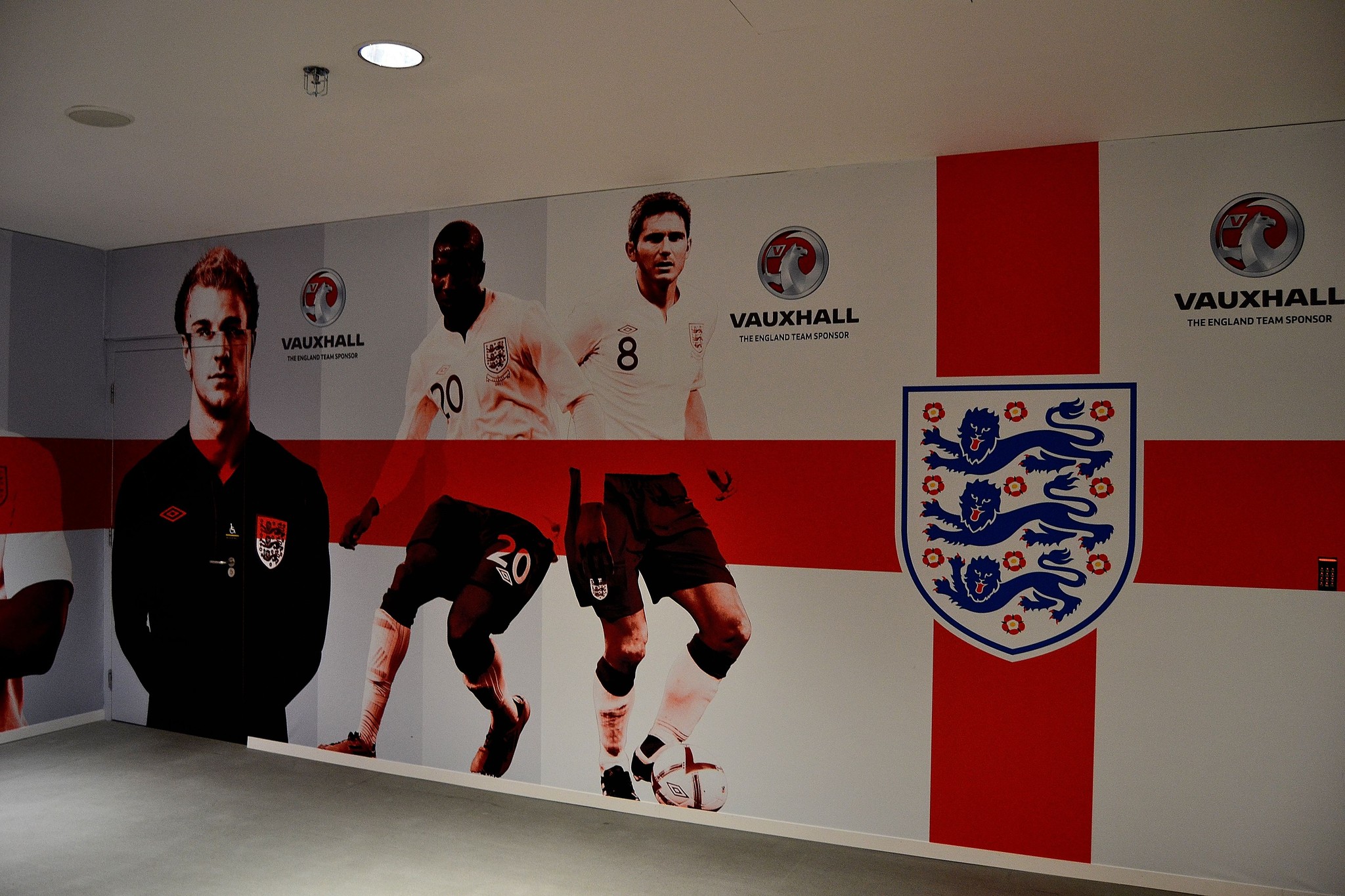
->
<box><xmin>317</xmin><ymin>220</ymin><xmax>613</xmax><ymax>778</ymax></box>
<box><xmin>0</xmin><ymin>430</ymin><xmax>74</xmax><ymax>733</ymax></box>
<box><xmin>112</xmin><ymin>246</ymin><xmax>332</xmax><ymax>743</ymax></box>
<box><xmin>563</xmin><ymin>191</ymin><xmax>751</xmax><ymax>803</ymax></box>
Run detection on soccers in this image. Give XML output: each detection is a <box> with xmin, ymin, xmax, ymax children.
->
<box><xmin>650</xmin><ymin>743</ymin><xmax>729</xmax><ymax>812</ymax></box>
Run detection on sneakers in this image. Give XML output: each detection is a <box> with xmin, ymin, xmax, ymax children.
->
<box><xmin>631</xmin><ymin>747</ymin><xmax>654</xmax><ymax>781</ymax></box>
<box><xmin>601</xmin><ymin>765</ymin><xmax>641</xmax><ymax>801</ymax></box>
<box><xmin>471</xmin><ymin>695</ymin><xmax>530</xmax><ymax>777</ymax></box>
<box><xmin>317</xmin><ymin>731</ymin><xmax>376</xmax><ymax>759</ymax></box>
